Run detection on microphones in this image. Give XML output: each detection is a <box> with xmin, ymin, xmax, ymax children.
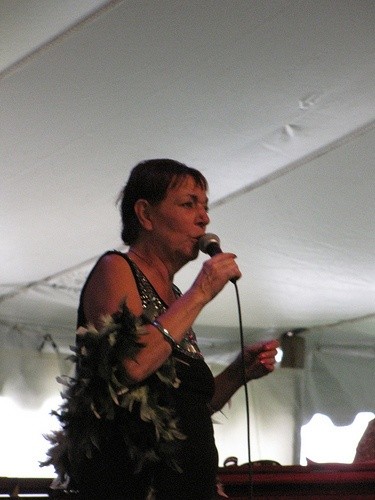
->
<box><xmin>198</xmin><ymin>233</ymin><xmax>237</xmax><ymax>282</ymax></box>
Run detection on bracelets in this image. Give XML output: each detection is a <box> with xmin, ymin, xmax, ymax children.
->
<box><xmin>152</xmin><ymin>320</ymin><xmax>178</xmax><ymax>351</ymax></box>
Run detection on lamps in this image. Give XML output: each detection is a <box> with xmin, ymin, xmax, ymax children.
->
<box><xmin>274</xmin><ymin>331</ymin><xmax>305</xmax><ymax>369</ymax></box>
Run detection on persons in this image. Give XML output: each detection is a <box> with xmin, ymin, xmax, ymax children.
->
<box><xmin>74</xmin><ymin>158</ymin><xmax>280</xmax><ymax>500</ymax></box>
